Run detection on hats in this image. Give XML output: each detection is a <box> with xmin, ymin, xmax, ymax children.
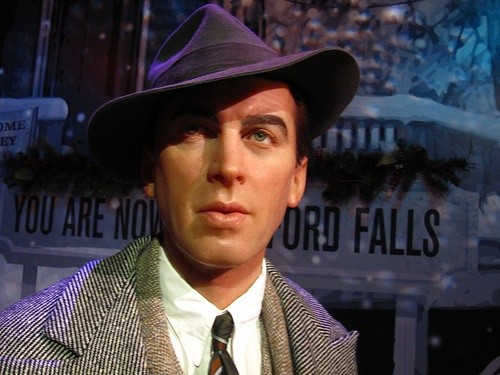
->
<box><xmin>85</xmin><ymin>4</ymin><xmax>360</xmax><ymax>191</ymax></box>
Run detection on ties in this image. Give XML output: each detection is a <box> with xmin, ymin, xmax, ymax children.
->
<box><xmin>207</xmin><ymin>313</ymin><xmax>239</xmax><ymax>375</ymax></box>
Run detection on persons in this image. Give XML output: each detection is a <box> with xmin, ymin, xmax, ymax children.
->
<box><xmin>0</xmin><ymin>4</ymin><xmax>361</xmax><ymax>375</ymax></box>
